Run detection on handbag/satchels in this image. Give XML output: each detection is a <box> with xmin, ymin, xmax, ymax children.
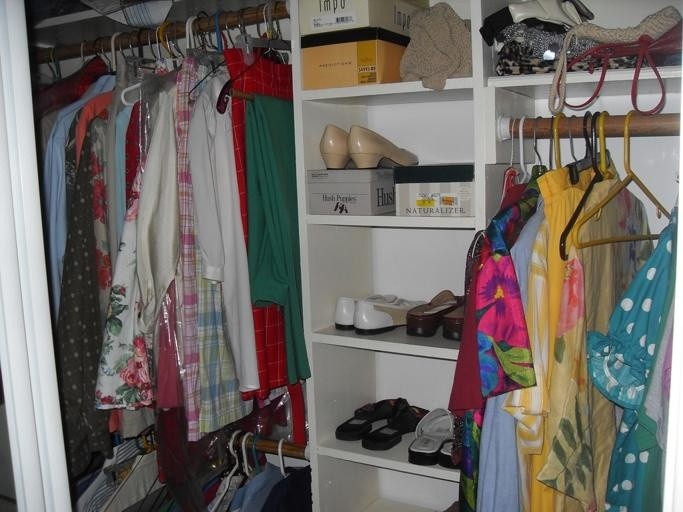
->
<box><xmin>548</xmin><ymin>5</ymin><xmax>682</xmax><ymax>116</ymax></box>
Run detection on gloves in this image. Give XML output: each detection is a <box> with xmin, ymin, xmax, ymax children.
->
<box><xmin>479</xmin><ymin>0</ymin><xmax>594</xmax><ymax>46</ymax></box>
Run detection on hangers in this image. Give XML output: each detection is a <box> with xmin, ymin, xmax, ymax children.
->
<box><xmin>497</xmin><ymin>108</ymin><xmax>673</xmax><ymax>262</ymax></box>
<box><xmin>37</xmin><ymin>2</ymin><xmax>291</xmax><ymax>108</ymax></box>
<box><xmin>107</xmin><ymin>428</ymin><xmax>294</xmax><ymax>512</ymax></box>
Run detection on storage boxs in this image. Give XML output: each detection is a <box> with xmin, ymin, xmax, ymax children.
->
<box><xmin>304</xmin><ymin>163</ymin><xmax>476</xmax><ymax>218</ymax></box>
<box><xmin>295</xmin><ymin>0</ymin><xmax>429</xmax><ymax>90</ymax></box>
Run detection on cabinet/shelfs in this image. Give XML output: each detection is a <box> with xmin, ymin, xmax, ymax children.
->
<box><xmin>289</xmin><ymin>0</ymin><xmax>683</xmax><ymax>512</ymax></box>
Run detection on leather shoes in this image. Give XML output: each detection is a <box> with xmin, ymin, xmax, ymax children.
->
<box><xmin>354</xmin><ymin>298</ymin><xmax>427</xmax><ymax>336</ymax></box>
<box><xmin>335</xmin><ymin>293</ymin><xmax>396</xmax><ymax>331</ymax></box>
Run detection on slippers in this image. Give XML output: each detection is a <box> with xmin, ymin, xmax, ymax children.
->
<box><xmin>442</xmin><ymin>306</ymin><xmax>465</xmax><ymax>341</ymax></box>
<box><xmin>361</xmin><ymin>404</ymin><xmax>429</xmax><ymax>449</ymax></box>
<box><xmin>408</xmin><ymin>408</ymin><xmax>459</xmax><ymax>469</ymax></box>
<box><xmin>335</xmin><ymin>398</ymin><xmax>397</xmax><ymax>441</ymax></box>
<box><xmin>406</xmin><ymin>290</ymin><xmax>465</xmax><ymax>337</ymax></box>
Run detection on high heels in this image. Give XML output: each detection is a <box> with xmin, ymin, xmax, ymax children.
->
<box><xmin>319</xmin><ymin>123</ymin><xmax>348</xmax><ymax>170</ymax></box>
<box><xmin>347</xmin><ymin>125</ymin><xmax>419</xmax><ymax>168</ymax></box>
